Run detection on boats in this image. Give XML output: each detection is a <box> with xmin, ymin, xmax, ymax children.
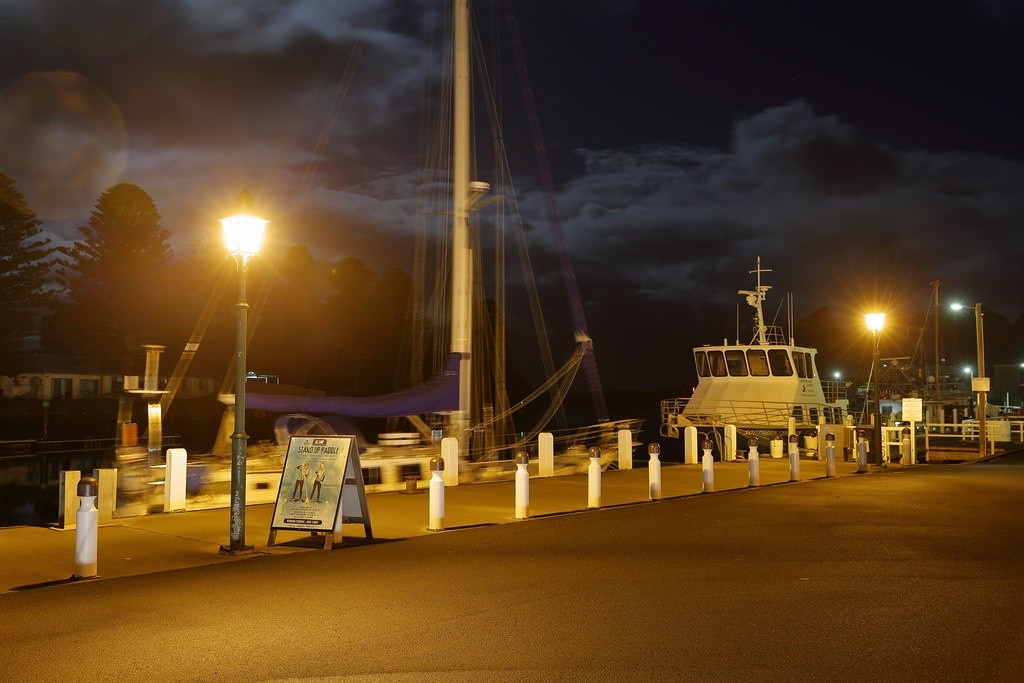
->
<box><xmin>660</xmin><ymin>255</ymin><xmax>856</xmax><ymax>457</ymax></box>
<box><xmin>856</xmin><ymin>279</ymin><xmax>1000</xmax><ymax>437</ymax></box>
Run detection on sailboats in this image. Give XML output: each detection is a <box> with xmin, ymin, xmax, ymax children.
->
<box><xmin>59</xmin><ymin>0</ymin><xmax>645</xmax><ymax>534</ymax></box>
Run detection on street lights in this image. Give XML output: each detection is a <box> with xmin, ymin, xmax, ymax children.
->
<box><xmin>218</xmin><ymin>186</ymin><xmax>272</xmax><ymax>558</ymax></box>
<box><xmin>863</xmin><ymin>300</ymin><xmax>887</xmax><ymax>470</ymax></box>
<box><xmin>949</xmin><ymin>302</ymin><xmax>987</xmax><ymax>458</ymax></box>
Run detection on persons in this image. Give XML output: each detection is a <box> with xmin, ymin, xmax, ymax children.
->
<box><xmin>291</xmin><ymin>463</ymin><xmax>325</xmax><ymax>503</ymax></box>
